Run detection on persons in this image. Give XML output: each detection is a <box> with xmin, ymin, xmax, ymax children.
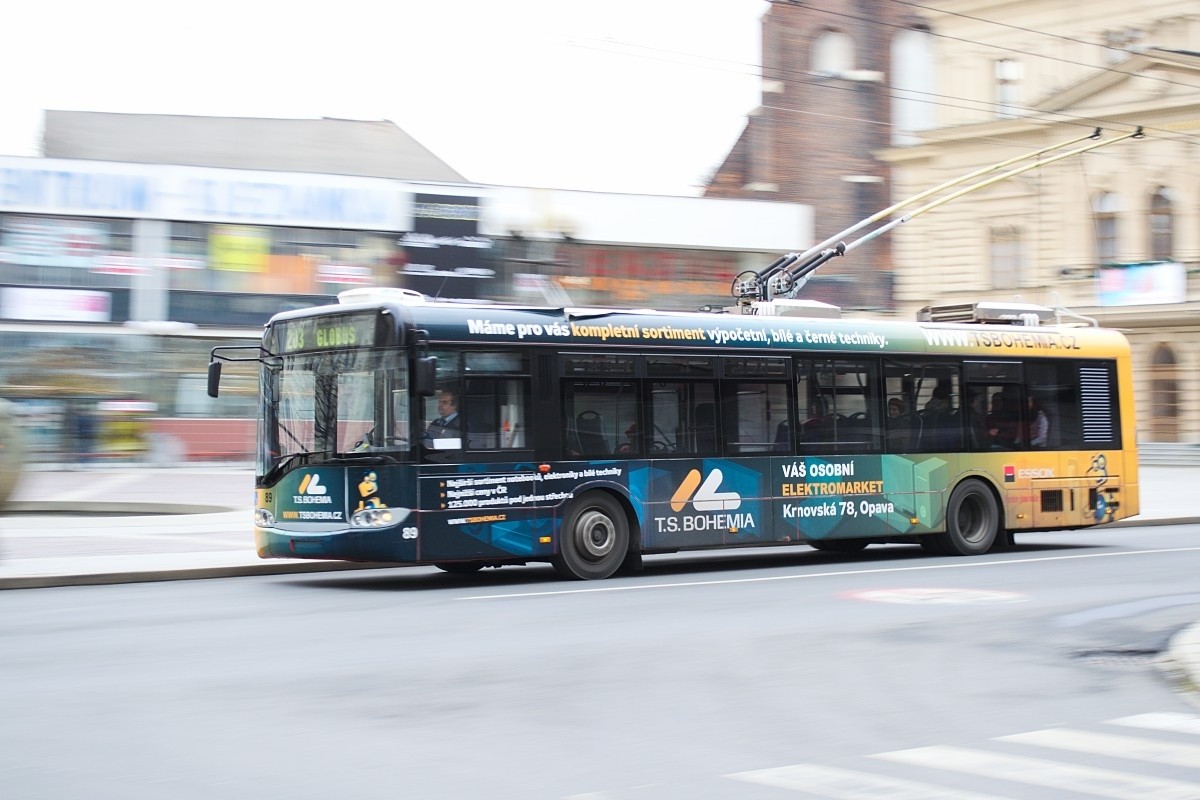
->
<box><xmin>1013</xmin><ymin>392</ymin><xmax>1050</xmax><ymax>449</ymax></box>
<box><xmin>619</xmin><ymin>424</ymin><xmax>638</xmax><ymax>456</ymax></box>
<box><xmin>950</xmin><ymin>390</ymin><xmax>1022</xmax><ymax>450</ymax></box>
<box><xmin>424</xmin><ymin>389</ymin><xmax>462</xmax><ymax>449</ymax></box>
<box><xmin>922</xmin><ymin>385</ymin><xmax>955</xmax><ymax>421</ymax></box>
<box><xmin>864</xmin><ymin>396</ymin><xmax>914</xmax><ymax>453</ymax></box>
<box><xmin>810</xmin><ymin>395</ymin><xmax>838</xmax><ymax>450</ymax></box>
<box><xmin>978</xmin><ymin>391</ymin><xmax>1016</xmax><ymax>446</ymax></box>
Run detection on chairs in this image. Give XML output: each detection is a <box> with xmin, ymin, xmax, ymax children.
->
<box><xmin>830</xmin><ymin>403</ymin><xmax>1059</xmax><ymax>457</ymax></box>
<box><xmin>772</xmin><ymin>420</ymin><xmax>803</xmax><ymax>453</ymax></box>
<box><xmin>574</xmin><ymin>401</ymin><xmax>728</xmax><ymax>455</ymax></box>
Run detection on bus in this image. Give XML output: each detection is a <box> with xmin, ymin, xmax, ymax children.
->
<box><xmin>207</xmin><ymin>113</ymin><xmax>1148</xmax><ymax>582</ymax></box>
